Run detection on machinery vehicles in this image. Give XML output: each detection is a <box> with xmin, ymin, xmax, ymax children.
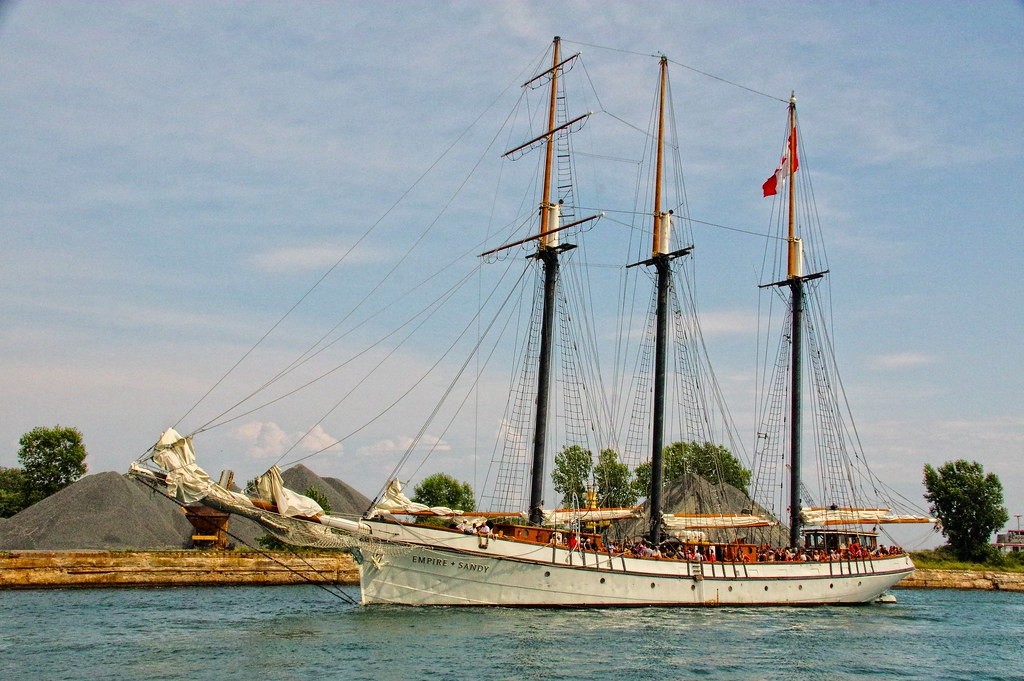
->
<box><xmin>181</xmin><ymin>468</ymin><xmax>235</xmax><ymax>552</ymax></box>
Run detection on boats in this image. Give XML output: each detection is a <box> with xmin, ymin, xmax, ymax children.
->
<box><xmin>874</xmin><ymin>594</ymin><xmax>899</xmax><ymax>604</ymax></box>
<box><xmin>989</xmin><ymin>514</ymin><xmax>1024</xmax><ymax>555</ymax></box>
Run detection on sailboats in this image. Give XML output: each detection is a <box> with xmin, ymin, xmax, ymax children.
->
<box><xmin>120</xmin><ymin>31</ymin><xmax>946</xmax><ymax>613</ymax></box>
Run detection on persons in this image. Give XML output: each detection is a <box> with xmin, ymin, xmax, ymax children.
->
<box><xmin>550</xmin><ymin>534</ymin><xmax>568</xmax><ymax>546</ymax></box>
<box><xmin>536</xmin><ymin>530</ymin><xmax>542</xmax><ymax>542</ymax></box>
<box><xmin>457</xmin><ymin>519</ymin><xmax>490</xmax><ymax>535</ymax></box>
<box><xmin>567</xmin><ymin>534</ymin><xmax>902</xmax><ymax>561</ymax></box>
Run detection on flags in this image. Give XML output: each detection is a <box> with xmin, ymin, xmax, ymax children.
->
<box><xmin>758</xmin><ymin>103</ymin><xmax>803</xmax><ymax>199</ymax></box>
